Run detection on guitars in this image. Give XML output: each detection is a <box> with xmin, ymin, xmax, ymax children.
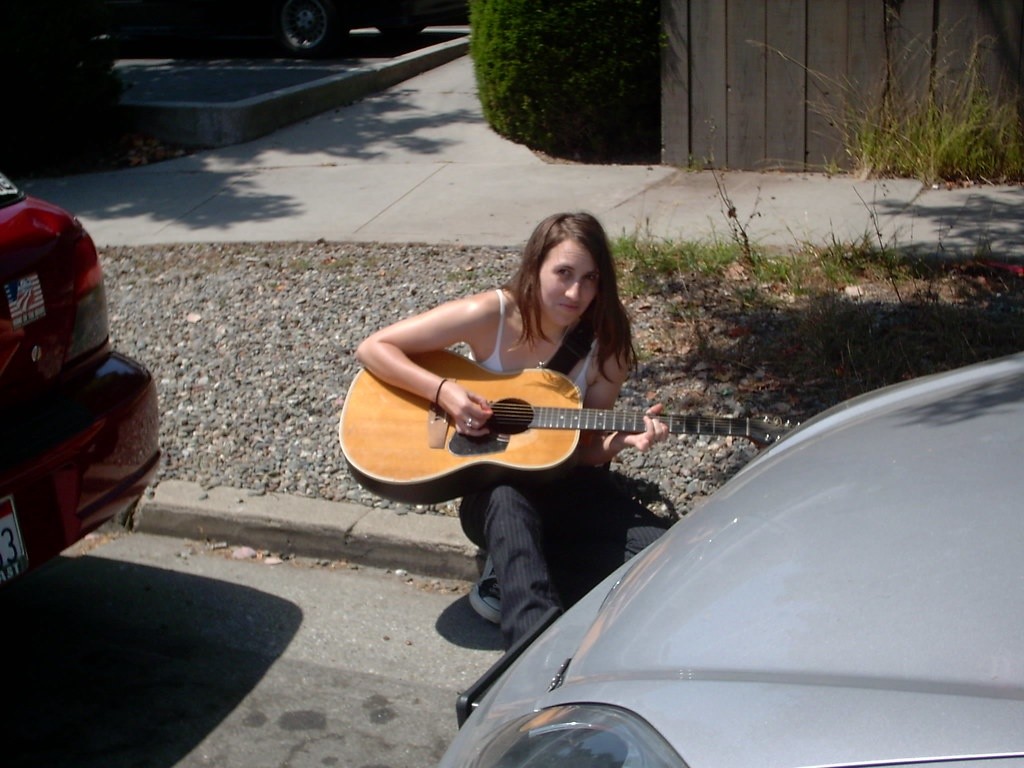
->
<box><xmin>335</xmin><ymin>344</ymin><xmax>800</xmax><ymax>508</ymax></box>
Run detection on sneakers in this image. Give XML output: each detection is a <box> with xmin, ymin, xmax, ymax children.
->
<box><xmin>469</xmin><ymin>552</ymin><xmax>505</xmax><ymax>625</ymax></box>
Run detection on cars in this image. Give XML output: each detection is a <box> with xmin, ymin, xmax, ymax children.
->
<box><xmin>0</xmin><ymin>168</ymin><xmax>164</xmax><ymax>600</ymax></box>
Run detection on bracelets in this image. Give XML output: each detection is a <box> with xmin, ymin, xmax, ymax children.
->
<box><xmin>436</xmin><ymin>379</ymin><xmax>446</xmax><ymax>404</ymax></box>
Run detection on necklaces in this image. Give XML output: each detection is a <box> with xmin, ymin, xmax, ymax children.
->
<box><xmin>535</xmin><ymin>331</ymin><xmax>567</xmax><ymax>369</ymax></box>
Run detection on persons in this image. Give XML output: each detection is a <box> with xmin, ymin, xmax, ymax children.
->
<box><xmin>356</xmin><ymin>212</ymin><xmax>669</xmax><ymax>653</ymax></box>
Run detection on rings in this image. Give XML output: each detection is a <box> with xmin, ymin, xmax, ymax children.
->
<box><xmin>467</xmin><ymin>418</ymin><xmax>472</xmax><ymax>427</ymax></box>
